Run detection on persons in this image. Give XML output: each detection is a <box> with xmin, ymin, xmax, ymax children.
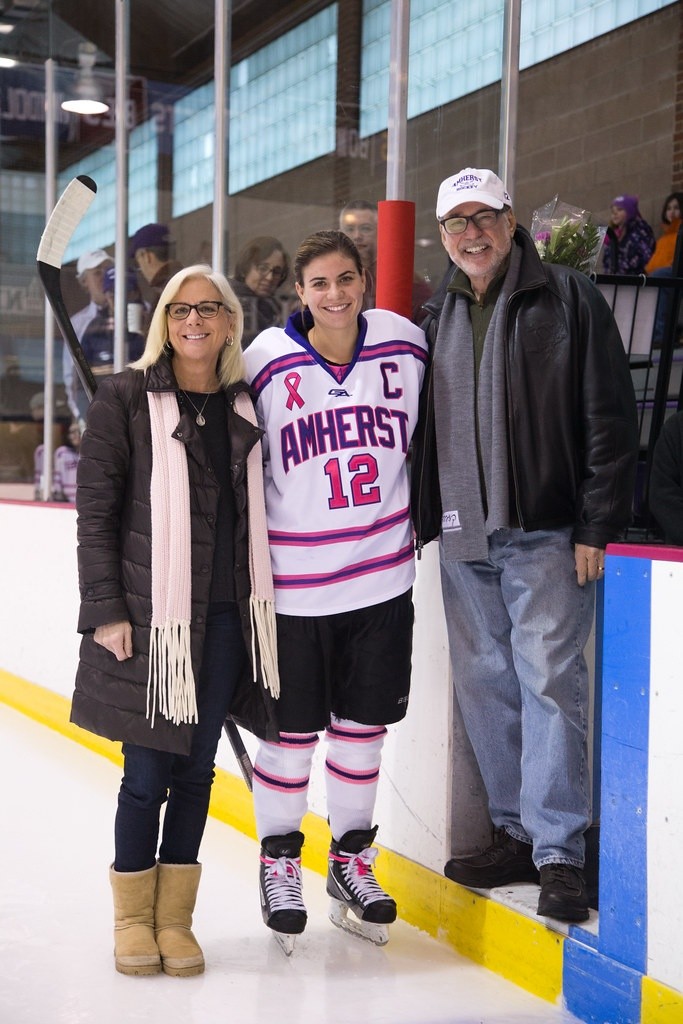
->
<box><xmin>240</xmin><ymin>231</ymin><xmax>430</xmax><ymax>958</ymax></box>
<box><xmin>35</xmin><ymin>170</ymin><xmax>682</xmax><ymax>545</ymax></box>
<box><xmin>403</xmin><ymin>169</ymin><xmax>637</xmax><ymax>930</ymax></box>
<box><xmin>73</xmin><ymin>265</ymin><xmax>280</xmax><ymax>977</ymax></box>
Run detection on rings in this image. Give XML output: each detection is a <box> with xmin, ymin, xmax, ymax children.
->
<box><xmin>598</xmin><ymin>566</ymin><xmax>605</xmax><ymax>570</ymax></box>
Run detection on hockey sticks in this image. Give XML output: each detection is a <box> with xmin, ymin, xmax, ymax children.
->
<box><xmin>36</xmin><ymin>175</ymin><xmax>255</xmax><ymax>791</ymax></box>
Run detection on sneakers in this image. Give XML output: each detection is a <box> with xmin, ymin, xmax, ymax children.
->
<box><xmin>326</xmin><ymin>824</ymin><xmax>397</xmax><ymax>946</ymax></box>
<box><xmin>259</xmin><ymin>831</ymin><xmax>308</xmax><ymax>957</ymax></box>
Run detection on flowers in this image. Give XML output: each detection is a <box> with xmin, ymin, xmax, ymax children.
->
<box><xmin>532</xmin><ymin>214</ymin><xmax>601</xmax><ymax>272</ymax></box>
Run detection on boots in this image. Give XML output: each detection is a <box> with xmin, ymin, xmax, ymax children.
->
<box><xmin>108</xmin><ymin>859</ymin><xmax>162</xmax><ymax>976</ymax></box>
<box><xmin>154</xmin><ymin>860</ymin><xmax>206</xmax><ymax>977</ymax></box>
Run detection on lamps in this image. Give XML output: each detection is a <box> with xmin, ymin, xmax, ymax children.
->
<box><xmin>60</xmin><ymin>42</ymin><xmax>112</xmax><ymax>115</ymax></box>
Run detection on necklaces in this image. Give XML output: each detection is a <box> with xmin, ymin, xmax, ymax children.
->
<box><xmin>176</xmin><ymin>380</ymin><xmax>212</xmax><ymax>426</ymax></box>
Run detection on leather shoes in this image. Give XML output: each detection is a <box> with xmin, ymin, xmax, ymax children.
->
<box><xmin>444</xmin><ymin>830</ymin><xmax>539</xmax><ymax>887</ymax></box>
<box><xmin>536</xmin><ymin>864</ymin><xmax>590</xmax><ymax>920</ymax></box>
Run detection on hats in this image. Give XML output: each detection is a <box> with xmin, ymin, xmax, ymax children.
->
<box><xmin>128</xmin><ymin>223</ymin><xmax>177</xmax><ymax>258</ymax></box>
<box><xmin>28</xmin><ymin>392</ymin><xmax>66</xmax><ymax>409</ymax></box>
<box><xmin>103</xmin><ymin>266</ymin><xmax>138</xmax><ymax>294</ymax></box>
<box><xmin>435</xmin><ymin>167</ymin><xmax>512</xmax><ymax>221</ymax></box>
<box><xmin>610</xmin><ymin>194</ymin><xmax>642</xmax><ymax>220</ymax></box>
<box><xmin>77</xmin><ymin>249</ymin><xmax>110</xmax><ymax>273</ymax></box>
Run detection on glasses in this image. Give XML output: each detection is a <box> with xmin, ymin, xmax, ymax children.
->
<box><xmin>254</xmin><ymin>263</ymin><xmax>284</xmax><ymax>280</ymax></box>
<box><xmin>165</xmin><ymin>301</ymin><xmax>233</xmax><ymax>321</ymax></box>
<box><xmin>439</xmin><ymin>205</ymin><xmax>511</xmax><ymax>235</ymax></box>
